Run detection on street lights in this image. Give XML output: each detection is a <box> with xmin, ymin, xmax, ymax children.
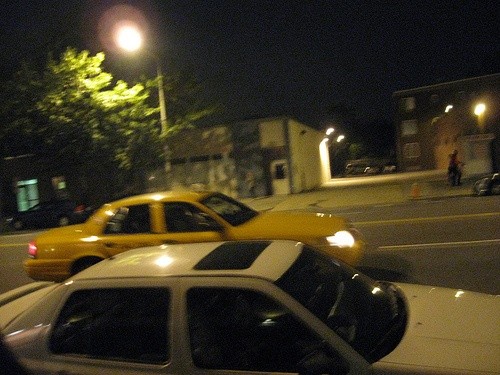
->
<box><xmin>113</xmin><ymin>21</ymin><xmax>173</xmax><ymax>193</ymax></box>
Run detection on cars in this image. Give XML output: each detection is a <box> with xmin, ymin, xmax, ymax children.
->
<box><xmin>7</xmin><ymin>197</ymin><xmax>93</xmax><ymax>231</ymax></box>
<box><xmin>1</xmin><ymin>238</ymin><xmax>500</xmax><ymax>375</ymax></box>
<box><xmin>23</xmin><ymin>186</ymin><xmax>364</xmax><ymax>282</ymax></box>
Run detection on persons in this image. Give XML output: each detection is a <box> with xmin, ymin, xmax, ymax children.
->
<box><xmin>448</xmin><ymin>150</ymin><xmax>463</xmax><ymax>187</ymax></box>
<box><xmin>246</xmin><ymin>172</ymin><xmax>259</xmax><ymax>200</ymax></box>
